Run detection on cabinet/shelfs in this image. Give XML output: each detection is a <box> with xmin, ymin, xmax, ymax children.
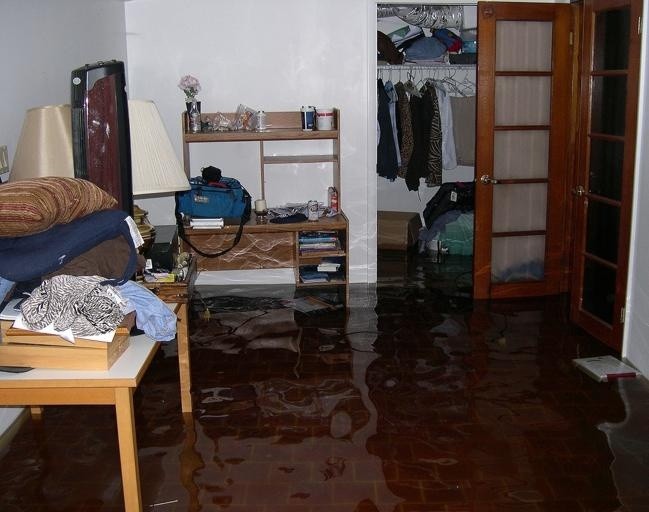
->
<box><xmin>178</xmin><ymin>108</ymin><xmax>349</xmax><ymax>305</ymax></box>
<box><xmin>143</xmin><ymin>224</ymin><xmax>179</xmax><ymax>272</ymax></box>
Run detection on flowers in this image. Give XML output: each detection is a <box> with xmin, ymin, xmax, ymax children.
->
<box><xmin>177</xmin><ymin>75</ymin><xmax>201</xmax><ymax>103</ymax></box>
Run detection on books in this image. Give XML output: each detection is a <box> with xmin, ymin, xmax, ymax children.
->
<box><xmin>299</xmin><ymin>230</ymin><xmax>344</xmax><ymax>256</ymax></box>
<box><xmin>300</xmin><ymin>262</ymin><xmax>347</xmax><ymax>283</ymax></box>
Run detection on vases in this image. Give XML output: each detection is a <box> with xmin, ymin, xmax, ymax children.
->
<box><xmin>186</xmin><ymin>102</ymin><xmax>201</xmax><ymax>133</ymax></box>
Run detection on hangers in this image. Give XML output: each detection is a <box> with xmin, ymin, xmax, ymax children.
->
<box><xmin>377</xmin><ymin>64</ymin><xmax>476</xmax><ymax>100</ymax></box>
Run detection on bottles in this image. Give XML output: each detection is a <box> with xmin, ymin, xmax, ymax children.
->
<box><xmin>189</xmin><ymin>99</ymin><xmax>202</xmax><ymax>132</ymax></box>
<box><xmin>256</xmin><ymin>111</ymin><xmax>266</xmax><ymax>131</ymax></box>
<box><xmin>316</xmin><ymin>105</ymin><xmax>334</xmax><ymax>130</ymax></box>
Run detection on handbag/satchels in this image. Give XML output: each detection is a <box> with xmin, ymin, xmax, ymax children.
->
<box><xmin>175</xmin><ymin>175</ymin><xmax>251</xmax><ymax>222</ymax></box>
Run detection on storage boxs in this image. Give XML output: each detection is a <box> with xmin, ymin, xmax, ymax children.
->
<box><xmin>377</xmin><ymin>211</ymin><xmax>422</xmax><ymax>250</ymax></box>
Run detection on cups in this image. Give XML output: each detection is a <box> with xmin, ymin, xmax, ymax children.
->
<box><xmin>301</xmin><ymin>108</ymin><xmax>315</xmax><ymax>131</ymax></box>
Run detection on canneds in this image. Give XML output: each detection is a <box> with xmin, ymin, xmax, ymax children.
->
<box><xmin>308</xmin><ymin>200</ymin><xmax>318</xmax><ymax>220</ymax></box>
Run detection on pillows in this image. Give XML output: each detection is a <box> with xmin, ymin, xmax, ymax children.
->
<box><xmin>0</xmin><ymin>177</ymin><xmax>119</xmax><ymax>237</ymax></box>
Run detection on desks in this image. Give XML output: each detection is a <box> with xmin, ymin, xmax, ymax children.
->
<box><xmin>0</xmin><ymin>293</ymin><xmax>192</xmax><ymax>512</ymax></box>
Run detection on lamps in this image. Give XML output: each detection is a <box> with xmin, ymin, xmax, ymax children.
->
<box><xmin>8</xmin><ymin>103</ymin><xmax>73</xmax><ymax>181</ymax></box>
<box><xmin>126</xmin><ymin>101</ymin><xmax>192</xmax><ymax>196</ymax></box>
